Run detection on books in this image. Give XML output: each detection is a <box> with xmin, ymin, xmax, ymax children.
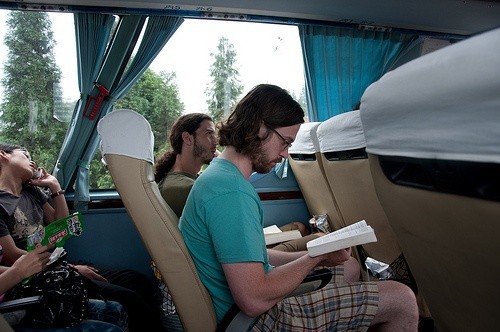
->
<box><xmin>306</xmin><ymin>219</ymin><xmax>377</xmax><ymax>257</ymax></box>
<box><xmin>261</xmin><ymin>225</ymin><xmax>301</xmax><ymax>247</ymax></box>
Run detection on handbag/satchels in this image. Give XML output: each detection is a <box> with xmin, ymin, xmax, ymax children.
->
<box><xmin>6</xmin><ymin>257</ymin><xmax>90</xmax><ymax>332</ymax></box>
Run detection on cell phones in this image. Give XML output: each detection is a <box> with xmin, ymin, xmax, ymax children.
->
<box><xmin>32</xmin><ymin>169</ymin><xmax>42</xmax><ymax>179</ymax></box>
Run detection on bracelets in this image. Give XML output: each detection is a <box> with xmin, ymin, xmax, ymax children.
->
<box><xmin>50</xmin><ymin>188</ymin><xmax>65</xmax><ymax>199</ymax></box>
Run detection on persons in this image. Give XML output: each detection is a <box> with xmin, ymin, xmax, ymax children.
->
<box><xmin>178</xmin><ymin>85</ymin><xmax>420</xmax><ymax>332</ymax></box>
<box><xmin>153</xmin><ymin>113</ymin><xmax>351</xmax><ymax>256</ymax></box>
<box><xmin>0</xmin><ymin>146</ymin><xmax>163</xmax><ymax>332</ymax></box>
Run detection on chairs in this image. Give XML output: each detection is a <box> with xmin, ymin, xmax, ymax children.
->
<box><xmin>97</xmin><ymin>109</ymin><xmax>334</xmax><ymax>332</ymax></box>
<box><xmin>287</xmin><ymin>56</ymin><xmax>500</xmax><ymax>332</ymax></box>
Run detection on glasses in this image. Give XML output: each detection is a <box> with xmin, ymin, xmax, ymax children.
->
<box><xmin>14</xmin><ymin>148</ymin><xmax>32</xmax><ymax>160</ymax></box>
<box><xmin>266</xmin><ymin>124</ymin><xmax>292</xmax><ymax>150</ymax></box>
